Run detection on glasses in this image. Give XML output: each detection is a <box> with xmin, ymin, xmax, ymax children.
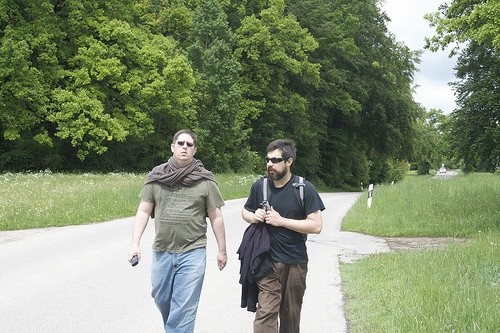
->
<box><xmin>266</xmin><ymin>157</ymin><xmax>285</xmax><ymax>163</ymax></box>
<box><xmin>175</xmin><ymin>140</ymin><xmax>194</xmax><ymax>147</ymax></box>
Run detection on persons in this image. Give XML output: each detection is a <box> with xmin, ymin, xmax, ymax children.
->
<box><xmin>241</xmin><ymin>138</ymin><xmax>323</xmax><ymax>333</ymax></box>
<box><xmin>127</xmin><ymin>128</ymin><xmax>228</xmax><ymax>333</ymax></box>
